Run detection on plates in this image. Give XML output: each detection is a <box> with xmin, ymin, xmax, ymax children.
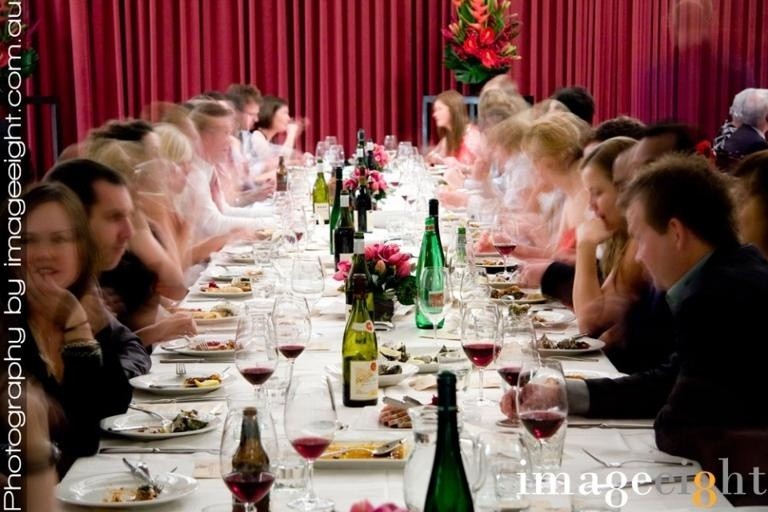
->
<box><xmin>50</xmin><ymin>473</ymin><xmax>195</xmax><ymax>508</ymax></box>
<box><xmin>159</xmin><ymin>243</ymin><xmax>259</xmax><ymax>354</ymax></box>
<box><xmin>302</xmin><ymin>431</ymin><xmax>408</xmax><ymax>470</ymax></box>
<box><xmin>99</xmin><ymin>411</ymin><xmax>223</xmax><ymax>441</ymax></box>
<box><xmin>475</xmin><ymin>255</ymin><xmax>574</xmax><ymax>326</ymax></box>
<box><xmin>519</xmin><ymin>334</ymin><xmax>606</xmax><ymax>357</ymax></box>
<box><xmin>321</xmin><ymin>359</ymin><xmax>420</xmax><ymax>388</ymax></box>
<box><xmin>126</xmin><ymin>369</ymin><xmax>232</xmax><ymax>394</ymax></box>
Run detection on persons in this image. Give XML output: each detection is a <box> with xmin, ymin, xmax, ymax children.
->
<box><xmin>422</xmin><ymin>73</ymin><xmax>768</xmax><ymax>505</ymax></box>
<box><xmin>0</xmin><ymin>81</ymin><xmax>311</xmax><ymax>511</ymax></box>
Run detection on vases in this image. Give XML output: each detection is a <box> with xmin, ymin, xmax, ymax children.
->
<box><xmin>371</xmin><ymin>300</ymin><xmax>393</xmax><ymax>320</ymax></box>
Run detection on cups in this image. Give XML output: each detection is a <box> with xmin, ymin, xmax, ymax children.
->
<box><xmin>518</xmin><ymin>418</ymin><xmax>568</xmax><ymax>482</ymax></box>
<box><xmin>436</xmin><ymin>349</ymin><xmax>474</xmax><ymax>391</ymax></box>
<box><xmin>403</xmin><ymin>405</ymin><xmax>463</xmax><ymax>445</ymax></box>
<box><xmin>402</xmin><ymin>428</ymin><xmax>537</xmax><ymax>511</ymax></box>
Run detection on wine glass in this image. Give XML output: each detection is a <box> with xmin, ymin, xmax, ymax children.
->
<box><xmin>218</xmin><ymin>405</ymin><xmax>277</xmax><ymax>512</ymax></box>
<box><xmin>232</xmin><ymin>134</ymin><xmax>345</xmax><ymax>400</ymax></box>
<box><xmin>382</xmin><ymin>133</ymin><xmax>540</xmax><ymax>427</ymax></box>
<box><xmin>282</xmin><ymin>371</ymin><xmax>339</xmax><ymax>511</ymax></box>
<box><xmin>513</xmin><ymin>357</ymin><xmax>570</xmax><ymax>488</ymax></box>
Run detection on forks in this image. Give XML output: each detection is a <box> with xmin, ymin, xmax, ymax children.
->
<box><xmin>579</xmin><ymin>445</ymin><xmax>694</xmax><ymax>469</ymax></box>
<box><xmin>122</xmin><ymin>403</ymin><xmax>173</xmax><ymax>428</ymax></box>
<box><xmin>180</xmin><ymin>325</ymin><xmax>207</xmax><ymax>350</ymax></box>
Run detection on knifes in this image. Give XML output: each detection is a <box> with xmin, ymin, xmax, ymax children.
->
<box><xmin>566</xmin><ymin>422</ymin><xmax>656</xmax><ymax>430</ymax></box>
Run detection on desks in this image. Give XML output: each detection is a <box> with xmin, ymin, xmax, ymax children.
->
<box><xmin>422</xmin><ymin>95</ymin><xmax>534</xmax><ymax>153</ymax></box>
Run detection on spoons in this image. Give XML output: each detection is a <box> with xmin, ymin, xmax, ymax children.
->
<box><xmin>315</xmin><ymin>437</ymin><xmax>409</xmax><ymax>460</ymax></box>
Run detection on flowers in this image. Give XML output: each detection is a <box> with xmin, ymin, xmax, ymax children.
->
<box><xmin>333</xmin><ymin>243</ymin><xmax>417</xmax><ymax>305</ymax></box>
<box><xmin>343</xmin><ymin>168</ymin><xmax>388</xmax><ymax>201</ymax></box>
<box><xmin>352</xmin><ymin>143</ymin><xmax>388</xmax><ymax>170</ymax></box>
<box><xmin>440</xmin><ymin>0</ymin><xmax>523</xmax><ymax>85</ymax></box>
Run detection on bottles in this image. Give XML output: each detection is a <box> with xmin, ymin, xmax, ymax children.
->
<box><xmin>413</xmin><ymin>198</ymin><xmax>447</xmax><ymax>328</ymax></box>
<box><xmin>311</xmin><ymin>129</ymin><xmax>379</xmax><ymax>408</ymax></box>
<box><xmin>420</xmin><ymin>369</ymin><xmax>475</xmax><ymax>512</ymax></box>
